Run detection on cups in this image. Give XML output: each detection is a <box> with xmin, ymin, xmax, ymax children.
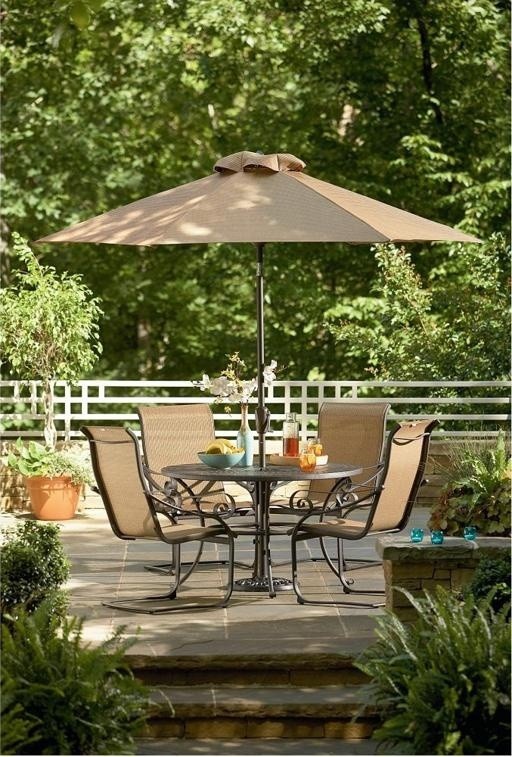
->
<box><xmin>300</xmin><ymin>454</ymin><xmax>316</xmax><ymax>473</ymax></box>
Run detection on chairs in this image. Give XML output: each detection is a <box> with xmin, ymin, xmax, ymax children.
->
<box><xmin>137</xmin><ymin>403</ymin><xmax>254</xmax><ymax>576</ymax></box>
<box><xmin>80</xmin><ymin>425</ymin><xmax>235</xmax><ymax>615</ymax></box>
<box><xmin>291</xmin><ymin>418</ymin><xmax>441</xmax><ymax>607</ymax></box>
<box><xmin>269</xmin><ymin>401</ymin><xmax>391</xmax><ymax>571</ymax></box>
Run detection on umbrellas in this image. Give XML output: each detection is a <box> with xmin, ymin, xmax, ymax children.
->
<box><xmin>36</xmin><ymin>152</ymin><xmax>485</xmax><ymax>591</ymax></box>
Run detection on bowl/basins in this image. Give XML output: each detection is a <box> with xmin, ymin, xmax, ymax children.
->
<box><xmin>198</xmin><ymin>453</ymin><xmax>244</xmax><ymax>469</ymax></box>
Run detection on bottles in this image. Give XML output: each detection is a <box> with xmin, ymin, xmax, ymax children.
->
<box><xmin>283</xmin><ymin>412</ymin><xmax>299</xmax><ymax>456</ymax></box>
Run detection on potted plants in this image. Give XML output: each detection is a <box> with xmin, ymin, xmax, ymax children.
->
<box><xmin>8</xmin><ymin>436</ymin><xmax>95</xmax><ymax>520</ymax></box>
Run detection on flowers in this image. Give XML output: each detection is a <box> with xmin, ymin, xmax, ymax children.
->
<box><xmin>190</xmin><ymin>350</ymin><xmax>290</xmax><ymax>417</ymax></box>
